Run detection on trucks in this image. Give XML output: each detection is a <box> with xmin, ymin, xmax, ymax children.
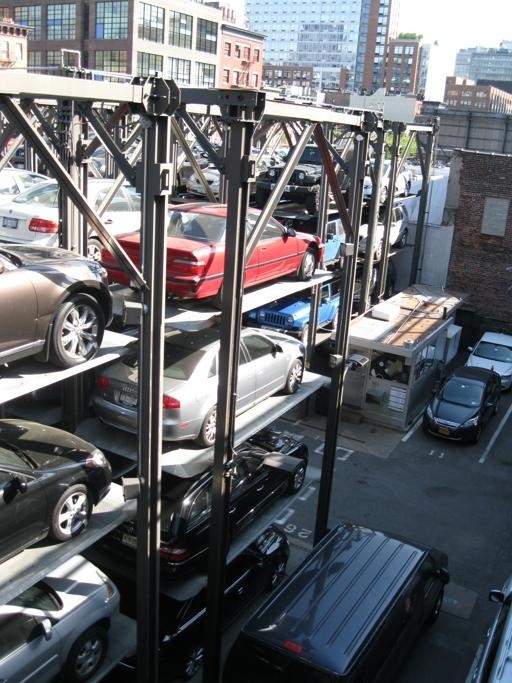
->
<box><xmin>221</xmin><ymin>522</ymin><xmax>449</xmax><ymax>683</ymax></box>
<box><xmin>462</xmin><ymin>572</ymin><xmax>511</xmax><ymax>682</ymax></box>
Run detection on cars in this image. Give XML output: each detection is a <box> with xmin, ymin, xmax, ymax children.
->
<box><xmin>422</xmin><ymin>364</ymin><xmax>501</xmax><ymax>444</ymax></box>
<box><xmin>465</xmin><ymin>329</ymin><xmax>511</xmax><ymax>391</ymax></box>
